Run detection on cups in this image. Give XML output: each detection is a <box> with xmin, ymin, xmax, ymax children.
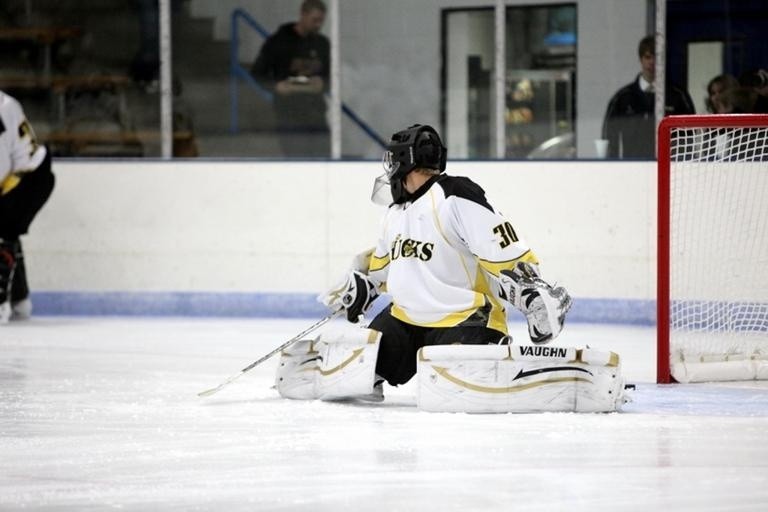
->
<box><xmin>714</xmin><ymin>134</ymin><xmax>732</xmax><ymax>159</ymax></box>
<box><xmin>595</xmin><ymin>139</ymin><xmax>610</xmax><ymax>158</ymax></box>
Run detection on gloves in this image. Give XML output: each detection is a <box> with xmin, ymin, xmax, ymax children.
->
<box><xmin>497</xmin><ymin>260</ymin><xmax>574</xmax><ymax>344</ymax></box>
<box><xmin>319</xmin><ymin>270</ymin><xmax>380</xmax><ymax>322</ymax></box>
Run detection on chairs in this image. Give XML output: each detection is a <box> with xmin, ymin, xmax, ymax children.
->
<box><xmin>2</xmin><ymin>2</ymin><xmax>200</xmax><ymax>161</ymax></box>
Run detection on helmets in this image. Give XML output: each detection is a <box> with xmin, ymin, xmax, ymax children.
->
<box><xmin>383</xmin><ymin>123</ymin><xmax>447</xmax><ymax>200</ymax></box>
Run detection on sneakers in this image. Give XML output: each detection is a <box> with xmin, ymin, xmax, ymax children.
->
<box><xmin>0</xmin><ymin>299</ymin><xmax>33</xmax><ymax>320</ymax></box>
<box><xmin>326</xmin><ymin>376</ymin><xmax>385</xmax><ymax>403</ymax></box>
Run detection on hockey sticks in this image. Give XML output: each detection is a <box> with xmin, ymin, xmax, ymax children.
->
<box><xmin>197</xmin><ymin>306</ymin><xmax>344</xmax><ymax>397</ymax></box>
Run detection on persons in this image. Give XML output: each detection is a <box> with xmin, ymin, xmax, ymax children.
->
<box><xmin>3</xmin><ymin>88</ymin><xmax>55</xmax><ymax>323</ymax></box>
<box><xmin>602</xmin><ymin>31</ymin><xmax>695</xmax><ymax>165</ymax></box>
<box><xmin>253</xmin><ymin>0</ymin><xmax>330</xmax><ymax>160</ymax></box>
<box><xmin>278</xmin><ymin>124</ymin><xmax>630</xmax><ymax>402</ymax></box>
<box><xmin>704</xmin><ymin>67</ymin><xmax>767</xmax><ymax>112</ymax></box>
